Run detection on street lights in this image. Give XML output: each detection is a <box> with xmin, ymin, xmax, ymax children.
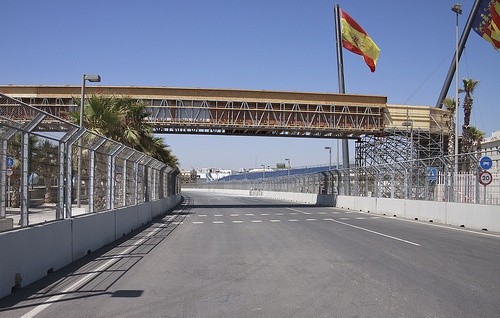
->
<box><xmin>285</xmin><ymin>159</ymin><xmax>290</xmax><ymax>177</ymax></box>
<box><xmin>243</xmin><ymin>169</ymin><xmax>246</xmax><ymax>179</ymax></box>
<box><xmin>325</xmin><ymin>147</ymin><xmax>331</xmax><ymax>171</ymax></box>
<box><xmin>261</xmin><ymin>165</ymin><xmax>265</xmax><ymax>179</ymax></box>
<box><xmin>76</xmin><ymin>74</ymin><xmax>101</xmax><ymax>209</ymax></box>
<box><xmin>452</xmin><ymin>3</ymin><xmax>463</xmax><ymax>202</ymax></box>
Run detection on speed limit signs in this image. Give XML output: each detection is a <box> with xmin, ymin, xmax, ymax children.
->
<box><xmin>478</xmin><ymin>171</ymin><xmax>492</xmax><ymax>185</ymax></box>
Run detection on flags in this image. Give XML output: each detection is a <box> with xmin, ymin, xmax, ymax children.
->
<box><xmin>338</xmin><ymin>7</ymin><xmax>382</xmax><ymax>73</ymax></box>
<box><xmin>470</xmin><ymin>0</ymin><xmax>500</xmax><ymax>50</ymax></box>
